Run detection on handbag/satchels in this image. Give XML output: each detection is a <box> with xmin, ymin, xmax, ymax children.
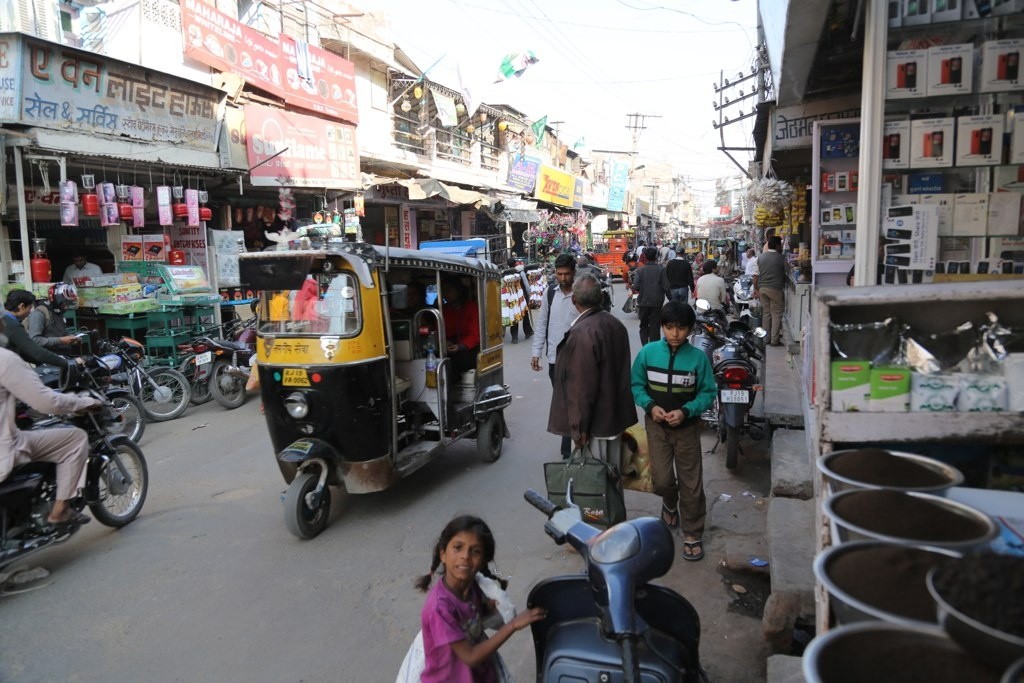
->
<box><xmin>246</xmin><ymin>363</ymin><xmax>261</xmax><ymax>393</ymax></box>
<box><xmin>624</xmin><ymin>422</ymin><xmax>656</xmax><ymax>493</ymax></box>
<box><xmin>543</xmin><ymin>444</ymin><xmax>627</xmax><ymax>526</ymax></box>
<box><xmin>621</xmin><ymin>292</ymin><xmax>634</xmax><ymax>313</ymax></box>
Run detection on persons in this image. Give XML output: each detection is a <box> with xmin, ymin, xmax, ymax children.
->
<box><xmin>846</xmin><ymin>236</ymin><xmax>892</xmax><ymax>288</ymax></box>
<box><xmin>0</xmin><ymin>347</ymin><xmax>101</xmax><ymax>526</ymax></box>
<box><xmin>27</xmin><ymin>282</ymin><xmax>84</xmax><ymax>357</ymax></box>
<box><xmin>64</xmin><ymin>253</ymin><xmax>102</xmax><ymax>285</ymax></box>
<box><xmin>413</xmin><ymin>515</ymin><xmax>546</xmax><ymax>683</ymax></box>
<box><xmin>530</xmin><ymin>254</ymin><xmax>581</xmax><ymax>460</ymax></box>
<box><xmin>547</xmin><ymin>273</ymin><xmax>639</xmax><ymax>554</ymax></box>
<box><xmin>630</xmin><ymin>300</ymin><xmax>716</xmax><ymax>562</ymax></box>
<box><xmin>0</xmin><ymin>288</ymin><xmax>86</xmax><ymax>370</ymax></box>
<box><xmin>552</xmin><ymin>228</ymin><xmax>790</xmax><ymax>347</ymax></box>
<box><xmin>502</xmin><ymin>257</ymin><xmax>534</xmax><ymax>344</ymax></box>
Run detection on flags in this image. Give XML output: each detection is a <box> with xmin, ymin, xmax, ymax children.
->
<box><xmin>430</xmin><ymin>48</ymin><xmax>539</xmax><ymax>128</ymax></box>
<box><xmin>532</xmin><ymin>115</ymin><xmax>547</xmax><ymax>150</ymax></box>
<box><xmin>573</xmin><ymin>135</ymin><xmax>584</xmax><ymax>149</ymax></box>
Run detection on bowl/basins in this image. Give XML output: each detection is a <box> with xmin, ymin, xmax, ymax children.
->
<box><xmin>800</xmin><ymin>620</ymin><xmax>1002</xmax><ymax>682</ymax></box>
<box><xmin>925</xmin><ymin>551</ymin><xmax>1024</xmax><ymax>666</ymax></box>
<box><xmin>815</xmin><ymin>448</ymin><xmax>965</xmax><ymax>498</ymax></box>
<box><xmin>813</xmin><ymin>538</ymin><xmax>964</xmax><ymax>632</ymax></box>
<box><xmin>1001</xmin><ymin>655</ymin><xmax>1024</xmax><ymax>683</ymax></box>
<box><xmin>820</xmin><ymin>488</ymin><xmax>1002</xmax><ymax>550</ymax></box>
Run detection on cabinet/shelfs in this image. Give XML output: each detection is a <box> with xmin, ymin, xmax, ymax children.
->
<box><xmin>58</xmin><ymin>304</ymin><xmax>219</xmax><ymax>389</ymax></box>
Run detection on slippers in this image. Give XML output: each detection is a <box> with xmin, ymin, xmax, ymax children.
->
<box><xmin>48</xmin><ymin>510</ymin><xmax>91</xmax><ymax>527</ymax></box>
<box><xmin>682</xmin><ymin>536</ymin><xmax>704</xmax><ymax>560</ymax></box>
<box><xmin>662</xmin><ymin>500</ymin><xmax>681</xmax><ymax>530</ymax></box>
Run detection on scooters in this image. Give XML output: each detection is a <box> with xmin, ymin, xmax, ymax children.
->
<box><xmin>524</xmin><ymin>478</ymin><xmax>710</xmax><ymax>683</ymax></box>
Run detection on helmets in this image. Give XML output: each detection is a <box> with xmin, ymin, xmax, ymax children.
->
<box><xmin>675</xmin><ymin>247</ymin><xmax>685</xmax><ymax>254</ymax></box>
<box><xmin>48</xmin><ymin>283</ymin><xmax>79</xmax><ymax>316</ymax></box>
<box><xmin>626</xmin><ymin>252</ymin><xmax>638</xmax><ymax>263</ymax></box>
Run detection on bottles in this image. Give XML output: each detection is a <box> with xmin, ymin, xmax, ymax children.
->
<box><xmin>425</xmin><ymin>348</ymin><xmax>437</xmax><ymax>371</ymax></box>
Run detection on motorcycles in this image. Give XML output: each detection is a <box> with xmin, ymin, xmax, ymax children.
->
<box><xmin>0</xmin><ymin>310</ymin><xmax>256</xmax><ymax>583</ymax></box>
<box><xmin>690</xmin><ymin>276</ymin><xmax>769</xmax><ymax>470</ymax></box>
<box><xmin>588</xmin><ymin>229</ymin><xmax>638</xmax><ymax>278</ymax></box>
<box><xmin>238</xmin><ymin>241</ymin><xmax>513</xmax><ymax>541</ymax></box>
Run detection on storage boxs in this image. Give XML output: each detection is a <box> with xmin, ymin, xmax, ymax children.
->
<box><xmin>819</xmin><ymin>171</ymin><xmax>858</xmax><ymax>258</ymax></box>
<box><xmin>829</xmin><ymin>357</ymin><xmax>1007</xmax><ymax>413</ymax></box>
<box><xmin>877</xmin><ymin>1</ymin><xmax>1024</xmax><ymax>268</ymax></box>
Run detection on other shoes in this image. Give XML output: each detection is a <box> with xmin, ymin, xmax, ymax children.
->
<box><xmin>771</xmin><ymin>341</ymin><xmax>784</xmax><ymax>346</ymax></box>
<box><xmin>511</xmin><ymin>338</ymin><xmax>517</xmax><ymax>344</ymax></box>
<box><xmin>764</xmin><ymin>340</ymin><xmax>771</xmax><ymax>344</ymax></box>
<box><xmin>525</xmin><ymin>331</ymin><xmax>533</xmax><ymax>339</ymax></box>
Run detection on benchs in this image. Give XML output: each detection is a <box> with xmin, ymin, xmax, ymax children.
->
<box><xmin>386</xmin><ymin>318</ymin><xmax>412</xmax><ymax>410</ymax></box>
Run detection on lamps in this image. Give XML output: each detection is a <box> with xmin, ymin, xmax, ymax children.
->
<box><xmin>491</xmin><ymin>199</ymin><xmax>503</xmax><ymax>214</ymax></box>
